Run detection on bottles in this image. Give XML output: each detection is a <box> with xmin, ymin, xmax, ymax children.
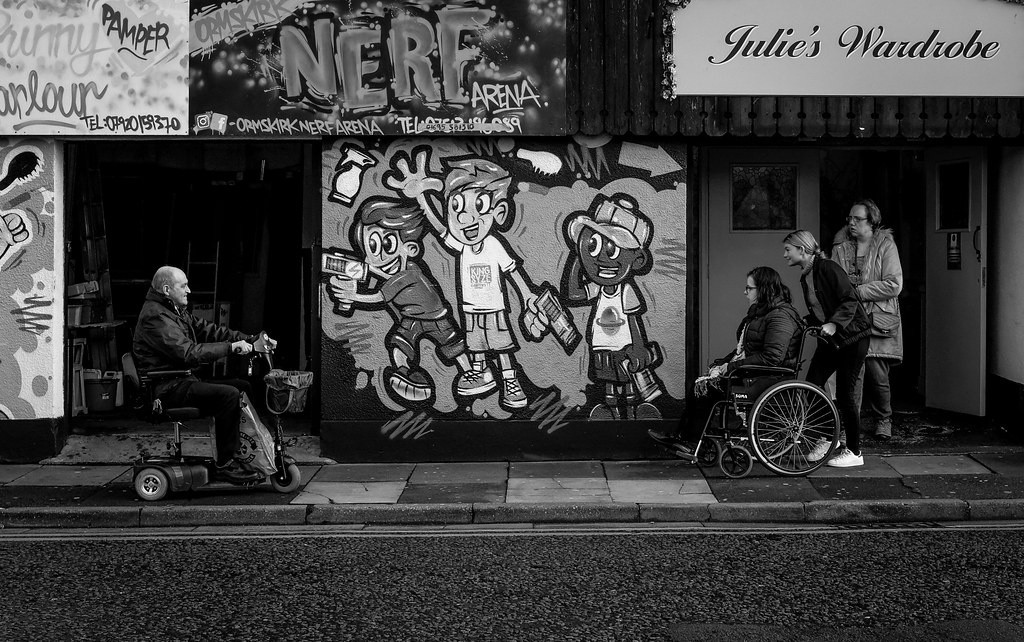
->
<box><xmin>103</xmin><ymin>372</ymin><xmax>123</xmax><ymax>406</ymax></box>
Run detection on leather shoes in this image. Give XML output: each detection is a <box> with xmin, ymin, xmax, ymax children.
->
<box><xmin>649</xmin><ymin>430</ymin><xmax>703</xmax><ymax>460</ymax></box>
<box><xmin>218</xmin><ymin>466</ymin><xmax>264</xmax><ymax>484</ymax></box>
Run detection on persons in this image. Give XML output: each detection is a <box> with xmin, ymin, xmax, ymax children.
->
<box><xmin>782</xmin><ymin>230</ymin><xmax>872</xmax><ymax>468</ymax></box>
<box><xmin>831</xmin><ymin>199</ymin><xmax>904</xmax><ymax>445</ymax></box>
<box><xmin>648</xmin><ymin>266</ymin><xmax>804</xmax><ymax>455</ymax></box>
<box><xmin>133</xmin><ymin>266</ymin><xmax>277</xmax><ymax>484</ymax></box>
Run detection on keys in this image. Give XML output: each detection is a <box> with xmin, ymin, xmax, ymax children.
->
<box><xmin>248</xmin><ymin>355</ymin><xmax>258</xmax><ymax>376</ymax></box>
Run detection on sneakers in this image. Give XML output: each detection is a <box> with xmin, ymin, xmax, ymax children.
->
<box><xmin>810</xmin><ymin>436</ymin><xmax>830</xmax><ymax>468</ymax></box>
<box><xmin>878</xmin><ymin>417</ymin><xmax>894</xmax><ymax>444</ymax></box>
<box><xmin>831</xmin><ymin>451</ymin><xmax>866</xmax><ymax>463</ymax></box>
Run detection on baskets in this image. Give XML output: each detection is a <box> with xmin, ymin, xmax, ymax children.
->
<box><xmin>262</xmin><ymin>367</ymin><xmax>314</xmax><ymax>414</ymax></box>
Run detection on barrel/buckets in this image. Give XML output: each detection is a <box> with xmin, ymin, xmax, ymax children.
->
<box><xmin>84</xmin><ymin>379</ymin><xmax>120</xmax><ymax>411</ymax></box>
<box><xmin>67</xmin><ymin>305</ymin><xmax>84</xmax><ymax>326</ymax></box>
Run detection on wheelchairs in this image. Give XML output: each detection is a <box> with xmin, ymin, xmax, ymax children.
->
<box><xmin>661</xmin><ymin>326</ymin><xmax>840</xmax><ymax>481</ymax></box>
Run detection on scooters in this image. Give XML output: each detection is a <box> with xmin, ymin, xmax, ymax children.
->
<box><xmin>121</xmin><ymin>329</ymin><xmax>314</xmax><ymax>501</ymax></box>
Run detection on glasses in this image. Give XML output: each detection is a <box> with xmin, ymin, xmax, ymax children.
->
<box><xmin>741</xmin><ymin>285</ymin><xmax>762</xmax><ymax>292</ymax></box>
<box><xmin>846</xmin><ymin>212</ymin><xmax>870</xmax><ymax>225</ymax></box>
